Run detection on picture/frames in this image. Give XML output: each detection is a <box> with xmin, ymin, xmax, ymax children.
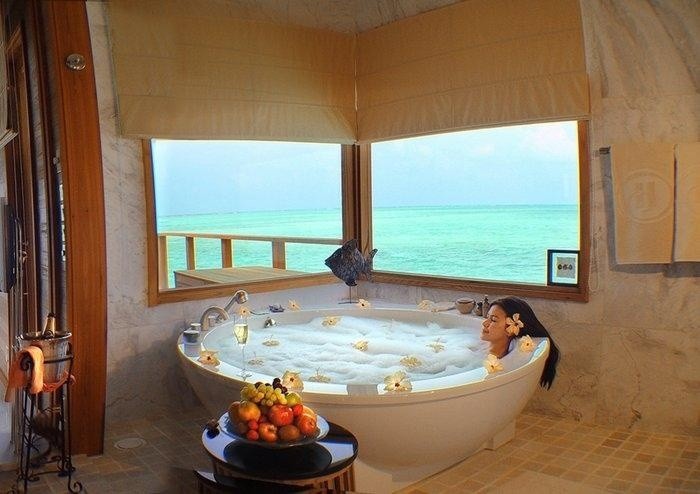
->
<box><xmin>543</xmin><ymin>247</ymin><xmax>580</xmax><ymax>289</ymax></box>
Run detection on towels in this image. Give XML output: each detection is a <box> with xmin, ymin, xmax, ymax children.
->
<box><xmin>606</xmin><ymin>140</ymin><xmax>685</xmax><ymax>268</ymax></box>
<box><xmin>668</xmin><ymin>139</ymin><xmax>700</xmax><ymax>266</ymax></box>
<box><xmin>6</xmin><ymin>344</ymin><xmax>43</xmax><ymax>394</ymax></box>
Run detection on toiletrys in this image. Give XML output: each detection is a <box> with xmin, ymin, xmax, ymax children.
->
<box><xmin>482</xmin><ymin>295</ymin><xmax>489</xmax><ymax>317</ymax></box>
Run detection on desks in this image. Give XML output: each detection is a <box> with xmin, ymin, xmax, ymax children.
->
<box><xmin>201</xmin><ymin>413</ymin><xmax>360</xmax><ymax>494</ymax></box>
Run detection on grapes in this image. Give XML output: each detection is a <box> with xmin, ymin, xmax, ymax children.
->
<box><xmin>241</xmin><ymin>377</ymin><xmax>288</xmax><ymax>406</ymax></box>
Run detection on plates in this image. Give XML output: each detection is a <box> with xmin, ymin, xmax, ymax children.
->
<box><xmin>223</xmin><ymin>439</ymin><xmax>331</xmax><ymax>475</ymax></box>
<box><xmin>216</xmin><ymin>403</ymin><xmax>330</xmax><ymax>451</ymax></box>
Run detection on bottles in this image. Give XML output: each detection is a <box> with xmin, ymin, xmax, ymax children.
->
<box><xmin>42</xmin><ymin>313</ymin><xmax>57</xmax><ymax>338</ymax></box>
<box><xmin>456</xmin><ymin>292</ymin><xmax>489</xmax><ymax>317</ymax></box>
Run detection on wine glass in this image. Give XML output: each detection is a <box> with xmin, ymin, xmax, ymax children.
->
<box><xmin>232</xmin><ymin>312</ymin><xmax>250</xmax><ymax>378</ymax></box>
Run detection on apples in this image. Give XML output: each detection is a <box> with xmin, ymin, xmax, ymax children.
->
<box><xmin>229</xmin><ymin>392</ymin><xmax>316</xmax><ymax>441</ymax></box>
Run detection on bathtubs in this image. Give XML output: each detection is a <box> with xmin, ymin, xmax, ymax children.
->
<box><xmin>176</xmin><ymin>299</ymin><xmax>550</xmax><ymax>494</ymax></box>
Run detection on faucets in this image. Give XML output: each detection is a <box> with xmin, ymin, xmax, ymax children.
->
<box><xmin>201</xmin><ymin>306</ymin><xmax>230</xmax><ymax>331</ymax></box>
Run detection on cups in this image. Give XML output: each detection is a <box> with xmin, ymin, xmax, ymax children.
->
<box><xmin>183</xmin><ymin>322</ymin><xmax>202</xmax><ymax>342</ymax></box>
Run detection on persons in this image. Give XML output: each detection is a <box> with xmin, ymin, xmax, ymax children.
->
<box><xmin>480</xmin><ymin>294</ymin><xmax>562</xmax><ymax>391</ymax></box>
